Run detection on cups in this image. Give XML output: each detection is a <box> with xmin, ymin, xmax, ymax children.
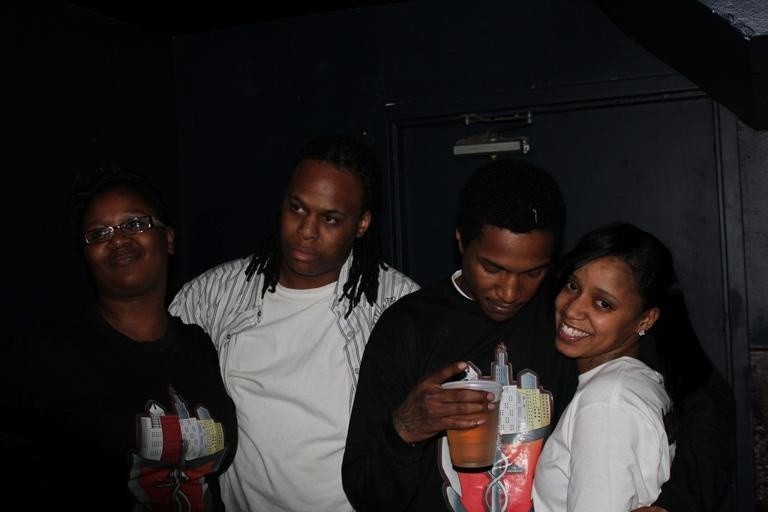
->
<box><xmin>441</xmin><ymin>380</ymin><xmax>502</xmax><ymax>474</ymax></box>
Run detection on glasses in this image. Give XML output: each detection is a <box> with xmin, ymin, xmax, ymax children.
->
<box><xmin>83</xmin><ymin>214</ymin><xmax>153</xmax><ymax>245</ymax></box>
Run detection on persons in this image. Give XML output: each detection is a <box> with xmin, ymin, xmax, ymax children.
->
<box><xmin>2</xmin><ymin>172</ymin><xmax>241</xmax><ymax>512</ymax></box>
<box><xmin>162</xmin><ymin>139</ymin><xmax>428</xmax><ymax>512</ymax></box>
<box><xmin>339</xmin><ymin>157</ymin><xmax>735</xmax><ymax>512</ymax></box>
<box><xmin>525</xmin><ymin>220</ymin><xmax>680</xmax><ymax>510</ymax></box>
